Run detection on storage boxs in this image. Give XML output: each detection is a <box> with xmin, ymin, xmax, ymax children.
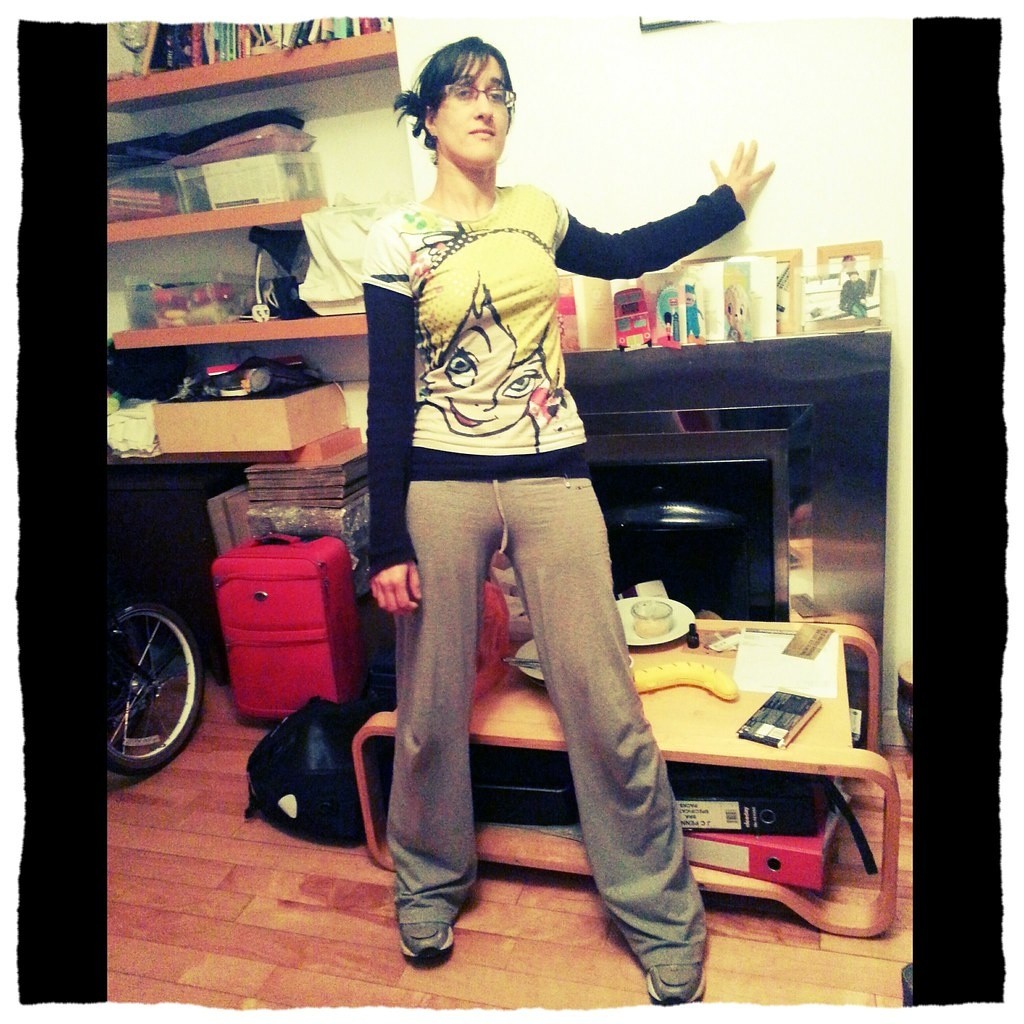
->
<box><xmin>152</xmin><ymin>382</ymin><xmax>349</xmax><ymax>453</ymax></box>
<box><xmin>124</xmin><ymin>273</ymin><xmax>257</xmax><ymax>329</ymax></box>
<box><xmin>173</xmin><ymin>150</ymin><xmax>325</xmax><ymax>214</ymax></box>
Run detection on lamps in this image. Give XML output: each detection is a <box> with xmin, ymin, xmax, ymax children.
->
<box><xmin>247</xmin><ymin>225</ymin><xmax>305</xmax><ymax>320</ymax></box>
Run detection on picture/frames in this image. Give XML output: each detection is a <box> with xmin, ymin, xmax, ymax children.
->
<box><xmin>813</xmin><ymin>240</ymin><xmax>883</xmax><ymax>333</ymax></box>
<box><xmin>640</xmin><ymin>248</ymin><xmax>800</xmax><ymax>341</ymax></box>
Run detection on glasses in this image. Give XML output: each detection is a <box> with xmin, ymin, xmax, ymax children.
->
<box><xmin>439</xmin><ymin>84</ymin><xmax>516</xmax><ymax>108</ymax></box>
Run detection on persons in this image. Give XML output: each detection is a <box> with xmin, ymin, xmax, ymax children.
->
<box><xmin>364</xmin><ymin>39</ymin><xmax>775</xmax><ymax>1001</ymax></box>
<box><xmin>838</xmin><ymin>272</ymin><xmax>867</xmax><ymax>319</ymax></box>
<box><xmin>841</xmin><ymin>255</ymin><xmax>858</xmax><ymax>272</ymax></box>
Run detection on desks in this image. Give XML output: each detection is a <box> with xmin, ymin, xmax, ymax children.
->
<box><xmin>351</xmin><ymin>618</ymin><xmax>899</xmax><ymax>940</ymax></box>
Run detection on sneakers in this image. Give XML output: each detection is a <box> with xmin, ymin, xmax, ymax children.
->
<box><xmin>645</xmin><ymin>961</ymin><xmax>706</xmax><ymax>1006</ymax></box>
<box><xmin>398</xmin><ymin>921</ymin><xmax>454</xmax><ymax>962</ymax></box>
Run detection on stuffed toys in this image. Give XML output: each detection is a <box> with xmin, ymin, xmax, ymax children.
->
<box><xmin>631</xmin><ymin>662</ymin><xmax>737</xmax><ymax>700</ymax></box>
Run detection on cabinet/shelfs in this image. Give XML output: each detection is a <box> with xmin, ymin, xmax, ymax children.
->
<box><xmin>106</xmin><ymin>28</ymin><xmax>398</xmax><ymax>464</ymax></box>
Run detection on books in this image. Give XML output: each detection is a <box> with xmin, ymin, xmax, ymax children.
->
<box><xmin>141</xmin><ymin>18</ymin><xmax>393</xmax><ymax>77</ymax></box>
<box><xmin>736</xmin><ymin>692</ymin><xmax>821</xmax><ymax>747</ymax></box>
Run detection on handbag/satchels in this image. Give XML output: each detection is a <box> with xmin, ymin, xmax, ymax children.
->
<box><xmin>243</xmin><ymin>695</ymin><xmax>386</xmax><ymax>849</ymax></box>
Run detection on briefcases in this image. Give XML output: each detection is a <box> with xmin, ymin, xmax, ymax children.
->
<box><xmin>210</xmin><ymin>532</ymin><xmax>363</xmax><ymax>725</ymax></box>
